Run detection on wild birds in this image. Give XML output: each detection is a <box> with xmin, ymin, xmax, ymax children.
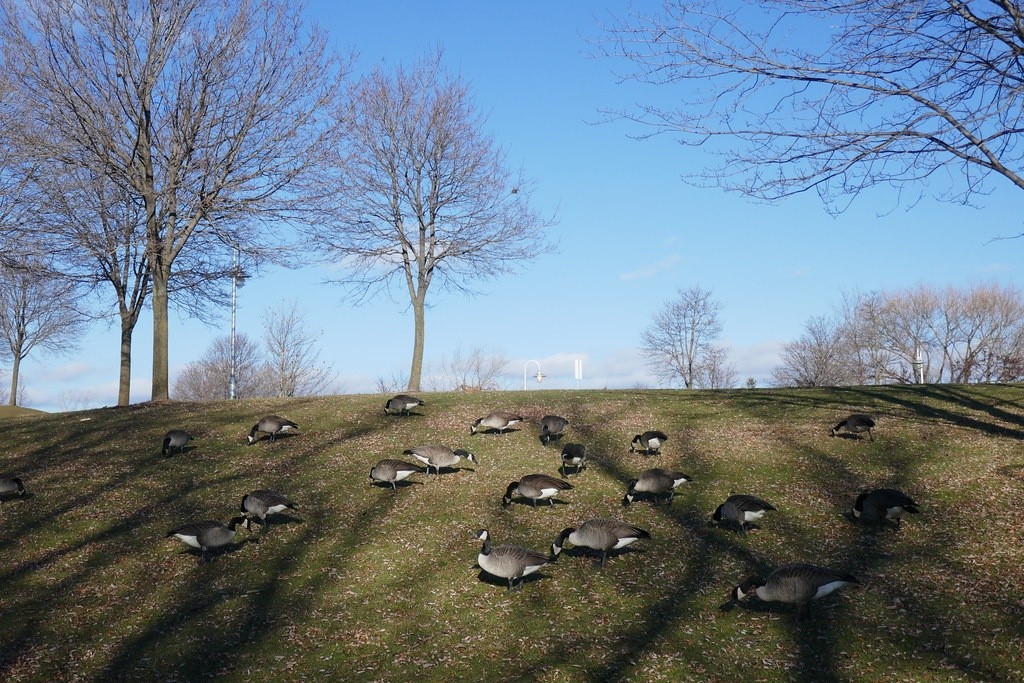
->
<box><xmin>469</xmin><ymin>411</ymin><xmax>523</xmax><ymax>439</ymax></box>
<box><xmin>852</xmin><ymin>487</ymin><xmax>921</xmax><ymax>536</ymax></box>
<box><xmin>0</xmin><ymin>474</ymin><xmax>27</xmax><ymax>500</ymax></box>
<box><xmin>502</xmin><ymin>474</ymin><xmax>575</xmax><ymax>513</ymax></box>
<box><xmin>540</xmin><ymin>414</ymin><xmax>570</xmax><ymax>448</ymax></box>
<box><xmin>728</xmin><ymin>562</ymin><xmax>861</xmax><ymax>624</ymax></box>
<box><xmin>630</xmin><ymin>430</ymin><xmax>668</xmax><ymax>457</ymax></box>
<box><xmin>384</xmin><ymin>394</ymin><xmax>427</xmax><ymax>418</ymax></box>
<box><xmin>560</xmin><ymin>442</ymin><xmax>587</xmax><ymax>479</ymax></box>
<box><xmin>624</xmin><ymin>467</ymin><xmax>694</xmax><ymax>508</ymax></box>
<box><xmin>246</xmin><ymin>415</ymin><xmax>300</xmax><ymax>444</ymax></box>
<box><xmin>161</xmin><ymin>429</ymin><xmax>194</xmax><ymax>458</ymax></box>
<box><xmin>368</xmin><ymin>459</ymin><xmax>427</xmax><ymax>496</ymax></box>
<box><xmin>831</xmin><ymin>413</ymin><xmax>876</xmax><ymax>443</ymax></box>
<box><xmin>469</xmin><ymin>529</ymin><xmax>560</xmax><ymax>594</ymax></box>
<box><xmin>400</xmin><ymin>444</ymin><xmax>478</xmax><ymax>479</ymax></box>
<box><xmin>163</xmin><ymin>488</ymin><xmax>300</xmax><ymax>564</ymax></box>
<box><xmin>709</xmin><ymin>495</ymin><xmax>778</xmax><ymax>539</ymax></box>
<box><xmin>549</xmin><ymin>517</ymin><xmax>652</xmax><ymax>571</ymax></box>
